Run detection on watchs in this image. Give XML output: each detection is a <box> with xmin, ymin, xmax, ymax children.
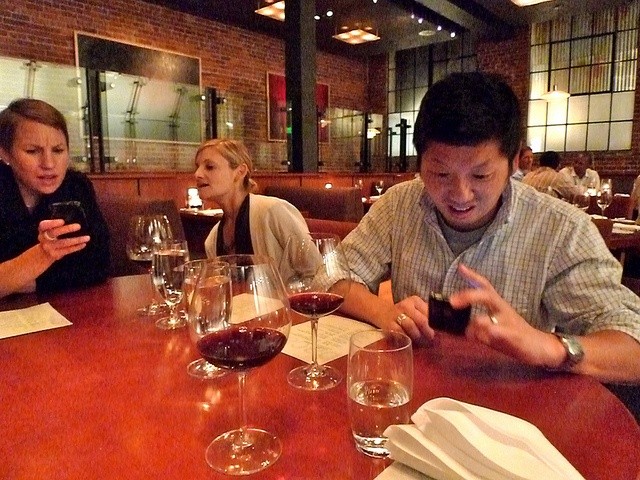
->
<box><xmin>542</xmin><ymin>332</ymin><xmax>584</xmax><ymax>374</ymax></box>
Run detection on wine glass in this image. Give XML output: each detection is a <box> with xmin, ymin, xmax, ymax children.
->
<box><xmin>601</xmin><ymin>179</ymin><xmax>613</xmax><ymax>188</ymax></box>
<box><xmin>188</xmin><ymin>253</ymin><xmax>293</xmax><ymax>477</ymax></box>
<box><xmin>126</xmin><ymin>215</ymin><xmax>175</xmax><ymax>316</ymax></box>
<box><xmin>151</xmin><ymin>240</ymin><xmax>190</xmax><ymax>331</ymax></box>
<box><xmin>375</xmin><ymin>180</ymin><xmax>385</xmax><ymax>197</ymax></box>
<box><xmin>573</xmin><ymin>188</ymin><xmax>591</xmax><ymax>213</ymax></box>
<box><xmin>182</xmin><ymin>259</ymin><xmax>233</xmax><ymax>380</ymax></box>
<box><xmin>275</xmin><ymin>233</ymin><xmax>351</xmax><ymax>392</ymax></box>
<box><xmin>596</xmin><ymin>188</ymin><xmax>613</xmax><ymax>219</ymax></box>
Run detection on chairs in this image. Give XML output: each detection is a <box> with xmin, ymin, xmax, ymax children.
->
<box><xmin>589</xmin><ymin>217</ymin><xmax>613</xmax><ymax>250</ymax></box>
<box><xmin>572</xmin><ymin>191</ymin><xmax>631</xmax><ymax>219</ymax></box>
<box><xmin>99</xmin><ymin>195</ymin><xmax>187</xmax><ymax>278</ymax></box>
<box><xmin>305</xmin><ymin>217</ymin><xmax>379</xmax><ymax>326</ymax></box>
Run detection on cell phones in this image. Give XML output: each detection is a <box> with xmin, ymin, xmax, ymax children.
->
<box><xmin>50</xmin><ymin>201</ymin><xmax>89</xmax><ymax>240</ymax></box>
<box><xmin>428</xmin><ymin>292</ymin><xmax>471</xmax><ymax>336</ymax></box>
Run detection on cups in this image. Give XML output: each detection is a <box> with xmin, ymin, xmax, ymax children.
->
<box><xmin>346</xmin><ymin>329</ymin><xmax>414</xmax><ymax>460</ymax></box>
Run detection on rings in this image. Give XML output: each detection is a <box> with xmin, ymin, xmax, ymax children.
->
<box><xmin>43</xmin><ymin>231</ymin><xmax>53</xmax><ymax>240</ymax></box>
<box><xmin>397</xmin><ymin>314</ymin><xmax>408</xmax><ymax>324</ymax></box>
<box><xmin>488</xmin><ymin>315</ymin><xmax>500</xmax><ymax>326</ymax></box>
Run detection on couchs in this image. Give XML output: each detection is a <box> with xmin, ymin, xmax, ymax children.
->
<box><xmin>263</xmin><ymin>186</ymin><xmax>365</xmax><ymax>223</ymax></box>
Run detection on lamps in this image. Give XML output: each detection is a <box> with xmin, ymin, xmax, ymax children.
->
<box><xmin>318</xmin><ymin>119</ymin><xmax>332</xmax><ymax>129</ymax></box>
<box><xmin>358</xmin><ymin>128</ymin><xmax>380</xmax><ymax>140</ymax></box>
<box><xmin>542</xmin><ymin>85</ymin><xmax>571</xmax><ymax>105</ymax></box>
<box><xmin>254</xmin><ymin>0</ymin><xmax>285</xmax><ymax>21</ymax></box>
<box><xmin>331</xmin><ymin>13</ymin><xmax>380</xmax><ymax>46</ymax></box>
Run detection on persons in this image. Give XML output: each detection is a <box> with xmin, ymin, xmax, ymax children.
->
<box><xmin>558</xmin><ymin>152</ymin><xmax>600</xmax><ymax>193</ymax></box>
<box><xmin>0</xmin><ymin>96</ymin><xmax>111</xmax><ymax>299</ymax></box>
<box><xmin>510</xmin><ymin>146</ymin><xmax>534</xmax><ymax>182</ymax></box>
<box><xmin>522</xmin><ymin>151</ymin><xmax>584</xmax><ymax>199</ymax></box>
<box><xmin>312</xmin><ymin>70</ymin><xmax>640</xmax><ymax>384</ymax></box>
<box><xmin>194</xmin><ymin>139</ymin><xmax>325</xmax><ymax>283</ymax></box>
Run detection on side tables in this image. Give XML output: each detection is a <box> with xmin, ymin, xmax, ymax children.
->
<box><xmin>180</xmin><ymin>207</ymin><xmax>225</xmax><ymax>254</ymax></box>
<box><xmin>584</xmin><ymin>214</ymin><xmax>640</xmax><ymax>269</ymax></box>
